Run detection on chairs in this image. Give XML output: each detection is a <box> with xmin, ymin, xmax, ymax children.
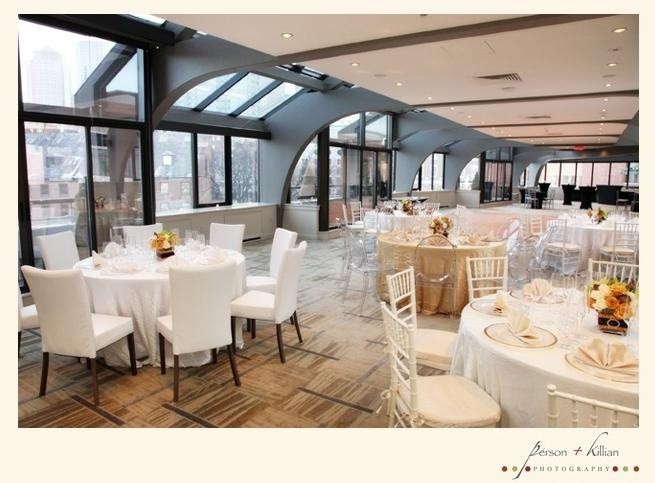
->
<box><xmin>36</xmin><ymin>230</ymin><xmax>80</xmax><ymax>272</ymax></box>
<box><xmin>245</xmin><ymin>227</ymin><xmax>301</xmax><ymax>333</ymax></box>
<box><xmin>230</xmin><ymin>240</ymin><xmax>309</xmax><ymax>364</ymax></box>
<box><xmin>152</xmin><ymin>258</ymin><xmax>241</xmax><ymax>404</ymax></box>
<box><xmin>121</xmin><ymin>222</ymin><xmax>164</xmax><ymax>250</ymax></box>
<box><xmin>207</xmin><ymin>221</ymin><xmax>246</xmax><ymax>253</ymax></box>
<box><xmin>19</xmin><ymin>264</ymin><xmax>137</xmax><ymax>407</ymax></box>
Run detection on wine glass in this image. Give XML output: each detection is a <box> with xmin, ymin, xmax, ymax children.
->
<box><xmin>101</xmin><ymin>228</ymin><xmax>216</xmax><ymax>273</ymax></box>
